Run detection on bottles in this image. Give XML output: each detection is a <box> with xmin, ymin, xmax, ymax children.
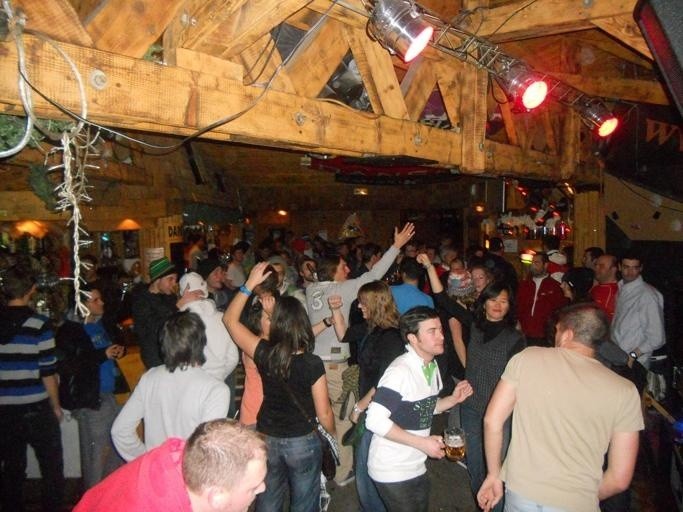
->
<box><xmin>305</xmin><ymin>262</ymin><xmax>319</xmax><ymax>281</ymax></box>
<box><xmin>541</xmin><ymin>226</ymin><xmax>546</xmax><ymax>239</ymax></box>
<box><xmin>531</xmin><ymin>225</ymin><xmax>537</xmax><ymax>240</ymax></box>
<box><xmin>552</xmin><ymin>226</ymin><xmax>556</xmax><ymax>237</ymax></box>
<box><xmin>559</xmin><ymin>225</ymin><xmax>565</xmax><ymax>240</ymax></box>
<box><xmin>41</xmin><ymin>308</ymin><xmax>50</xmax><ymax>318</ymax></box>
<box><xmin>35</xmin><ymin>306</ymin><xmax>41</xmax><ymax>315</ymax></box>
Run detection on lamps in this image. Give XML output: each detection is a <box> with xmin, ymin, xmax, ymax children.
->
<box><xmin>365</xmin><ymin>0</ymin><xmax>619</xmax><ymax>139</ymax></box>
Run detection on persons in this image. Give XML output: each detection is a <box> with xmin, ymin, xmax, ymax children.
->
<box><xmin>1</xmin><ymin>220</ymin><xmax>683</xmax><ymax>512</ymax></box>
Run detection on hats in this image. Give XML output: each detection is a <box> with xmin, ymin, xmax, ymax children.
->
<box><xmin>448</xmin><ymin>270</ymin><xmax>474</xmax><ymax>297</ymax></box>
<box><xmin>149</xmin><ymin>255</ymin><xmax>227</xmax><ymax>298</ymax></box>
<box><xmin>562</xmin><ymin>268</ymin><xmax>595</xmax><ymax>299</ymax></box>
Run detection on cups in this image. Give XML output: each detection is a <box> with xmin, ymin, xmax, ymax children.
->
<box><xmin>384</xmin><ymin>275</ymin><xmax>390</xmax><ymax>281</ymax></box>
<box><xmin>441</xmin><ymin>427</ymin><xmax>466</xmax><ymax>462</ymax></box>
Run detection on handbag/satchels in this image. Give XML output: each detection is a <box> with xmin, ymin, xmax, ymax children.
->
<box><xmin>315</xmin><ymin>418</ymin><xmax>340</xmax><ymax>480</ymax></box>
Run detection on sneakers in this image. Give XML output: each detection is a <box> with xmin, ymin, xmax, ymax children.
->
<box><xmin>337</xmin><ymin>468</ymin><xmax>355</xmax><ymax>486</ymax></box>
